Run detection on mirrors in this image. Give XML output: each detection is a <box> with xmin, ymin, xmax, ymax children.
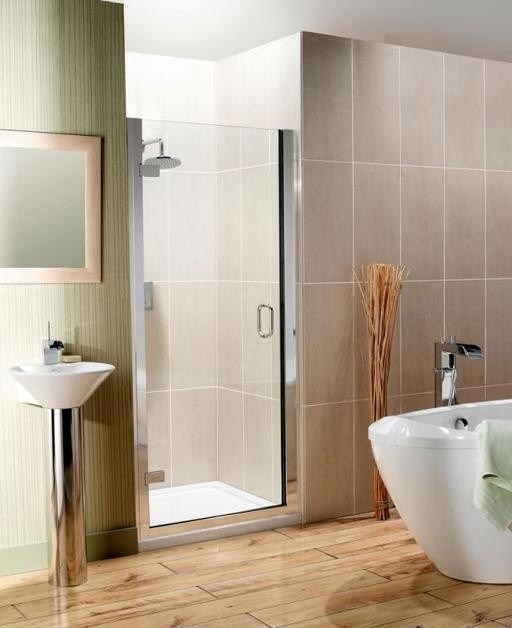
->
<box><xmin>0</xmin><ymin>130</ymin><xmax>102</xmax><ymax>283</ymax></box>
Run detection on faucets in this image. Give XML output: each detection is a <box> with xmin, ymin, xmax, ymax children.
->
<box><xmin>47</xmin><ymin>340</ymin><xmax>65</xmax><ymax>352</ymax></box>
<box><xmin>47</xmin><ymin>320</ymin><xmax>50</xmax><ymax>339</ymax></box>
<box><xmin>434</xmin><ymin>335</ymin><xmax>483</xmax><ymax>406</ymax></box>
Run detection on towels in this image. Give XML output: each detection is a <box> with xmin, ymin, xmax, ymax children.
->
<box><xmin>472</xmin><ymin>421</ymin><xmax>511</xmax><ymax>529</ymax></box>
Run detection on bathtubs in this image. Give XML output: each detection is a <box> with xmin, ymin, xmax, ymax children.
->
<box><xmin>368</xmin><ymin>397</ymin><xmax>512</xmax><ymax>585</ymax></box>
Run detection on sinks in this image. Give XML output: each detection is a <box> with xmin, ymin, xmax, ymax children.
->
<box><xmin>8</xmin><ymin>361</ymin><xmax>116</xmax><ymax>409</ymax></box>
